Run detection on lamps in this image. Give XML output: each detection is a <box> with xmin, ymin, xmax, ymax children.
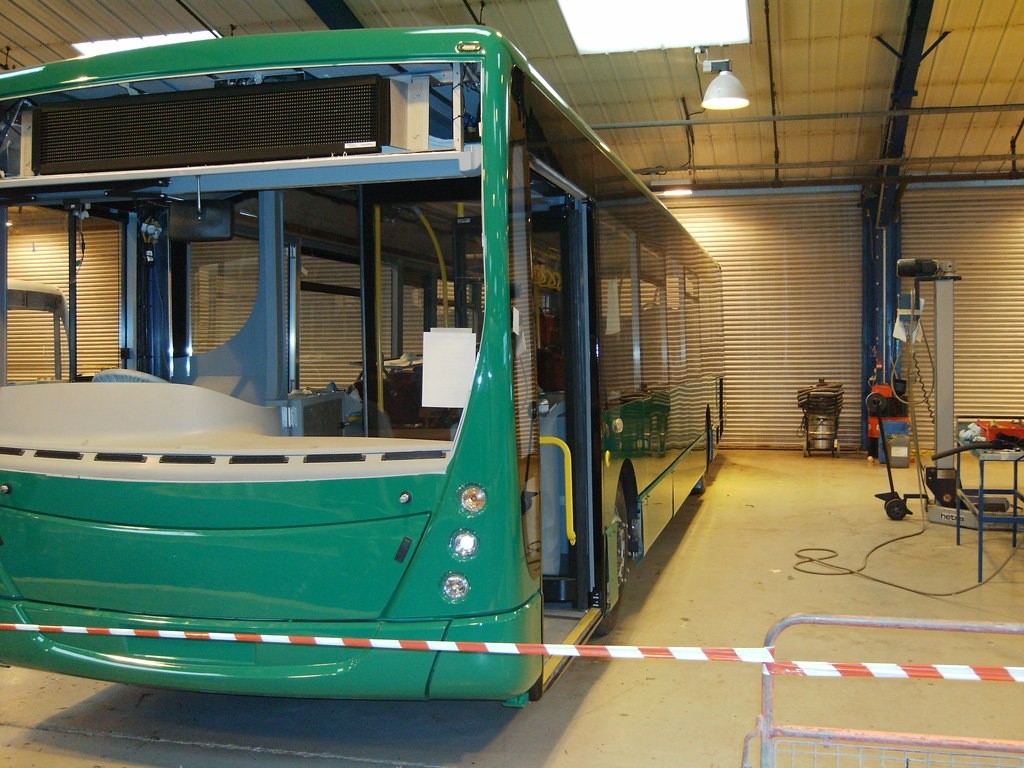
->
<box><xmin>700</xmin><ymin>60</ymin><xmax>750</xmax><ymax>111</ymax></box>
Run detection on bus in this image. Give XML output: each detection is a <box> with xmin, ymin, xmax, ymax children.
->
<box><xmin>0</xmin><ymin>24</ymin><xmax>726</xmax><ymax>709</ymax></box>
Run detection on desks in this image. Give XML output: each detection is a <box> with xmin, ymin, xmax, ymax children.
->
<box><xmin>954</xmin><ymin>421</ymin><xmax>1024</xmax><ymax>583</ymax></box>
